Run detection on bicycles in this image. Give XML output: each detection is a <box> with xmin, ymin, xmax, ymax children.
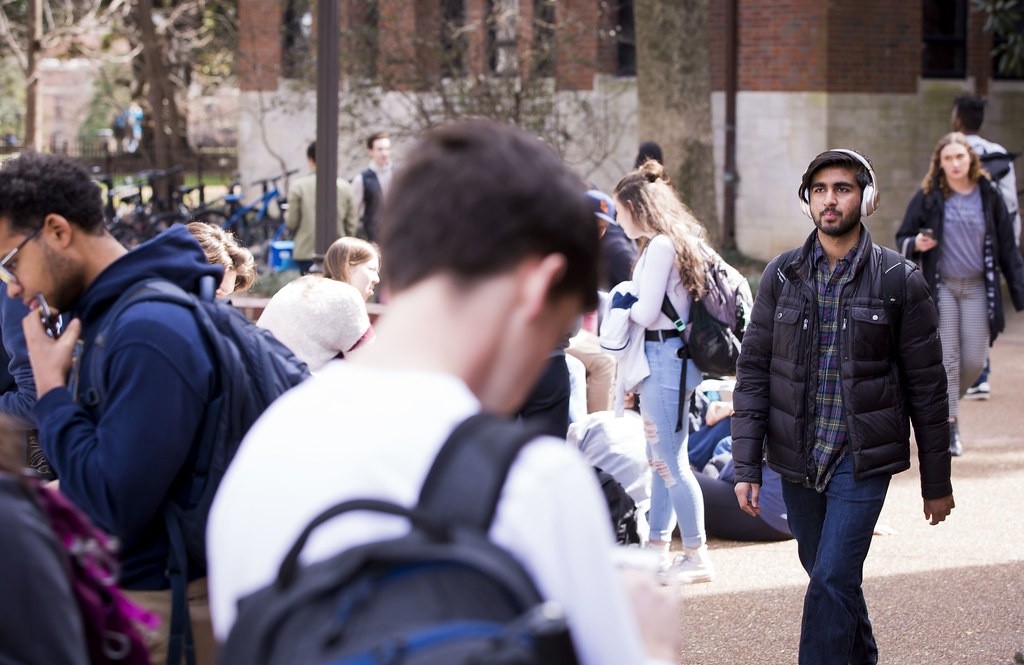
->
<box><xmin>103</xmin><ymin>167</ymin><xmax>298</xmax><ymax>249</ymax></box>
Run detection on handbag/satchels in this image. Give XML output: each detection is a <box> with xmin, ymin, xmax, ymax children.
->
<box><xmin>28</xmin><ymin>472</ymin><xmax>165</xmax><ymax>665</ymax></box>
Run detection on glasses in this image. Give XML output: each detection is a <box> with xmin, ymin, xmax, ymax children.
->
<box><xmin>0</xmin><ymin>218</ymin><xmax>46</xmax><ymax>283</ymax></box>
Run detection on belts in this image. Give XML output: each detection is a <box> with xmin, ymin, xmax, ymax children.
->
<box><xmin>644</xmin><ymin>328</ymin><xmax>681</xmax><ymax>342</ymax></box>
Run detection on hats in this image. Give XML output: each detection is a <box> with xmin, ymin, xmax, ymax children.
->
<box><xmin>583</xmin><ymin>189</ymin><xmax>618</xmax><ymax>226</ymax></box>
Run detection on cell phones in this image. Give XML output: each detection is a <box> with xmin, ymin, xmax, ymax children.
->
<box><xmin>918</xmin><ymin>229</ymin><xmax>934</xmax><ymax>241</ymax></box>
<box><xmin>37</xmin><ymin>292</ymin><xmax>58</xmax><ymax>341</ymax></box>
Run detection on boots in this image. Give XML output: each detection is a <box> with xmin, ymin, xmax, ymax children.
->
<box><xmin>656</xmin><ymin>546</ymin><xmax>710</xmax><ymax>586</ymax></box>
<box><xmin>641</xmin><ymin>540</ymin><xmax>670</xmax><ymax>573</ymax></box>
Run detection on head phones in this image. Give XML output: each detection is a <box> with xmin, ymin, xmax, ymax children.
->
<box><xmin>800</xmin><ymin>148</ymin><xmax>880</xmax><ymax>217</ymax></box>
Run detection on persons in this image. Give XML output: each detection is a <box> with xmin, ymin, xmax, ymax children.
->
<box><xmin>0</xmin><ymin>147</ymin><xmax>312</xmax><ymax>665</ymax></box>
<box><xmin>730</xmin><ymin>148</ymin><xmax>956</xmax><ymax>665</ymax></box>
<box><xmin>205</xmin><ymin>118</ymin><xmax>652</xmax><ymax>665</ymax></box>
<box><xmin>897</xmin><ymin>94</ymin><xmax>1024</xmax><ymax>455</ymax></box>
<box><xmin>255</xmin><ymin>133</ymin><xmax>793</xmax><ymax>585</ymax></box>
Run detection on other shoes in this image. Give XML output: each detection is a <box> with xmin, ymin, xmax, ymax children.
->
<box><xmin>964</xmin><ymin>381</ymin><xmax>990</xmax><ymax>401</ymax></box>
<box><xmin>949</xmin><ymin>417</ymin><xmax>962</xmax><ymax>455</ymax></box>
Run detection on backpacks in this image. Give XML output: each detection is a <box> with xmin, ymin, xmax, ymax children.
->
<box><xmin>93</xmin><ymin>281</ymin><xmax>311</xmax><ymax>485</ymax></box>
<box><xmin>661</xmin><ymin>250</ymin><xmax>753</xmax><ymax>378</ymax></box>
<box><xmin>223</xmin><ymin>414</ymin><xmax>581</xmax><ymax>665</ymax></box>
